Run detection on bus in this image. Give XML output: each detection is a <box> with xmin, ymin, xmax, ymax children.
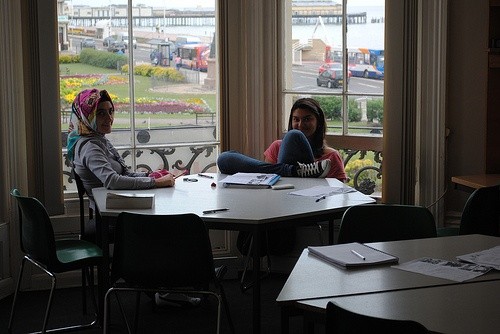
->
<box><xmin>327</xmin><ymin>48</ymin><xmax>384</xmax><ymax>80</ymax></box>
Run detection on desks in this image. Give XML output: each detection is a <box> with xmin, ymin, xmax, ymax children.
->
<box><xmin>276</xmin><ymin>233</ymin><xmax>500</xmax><ymax>334</ymax></box>
<box><xmin>92</xmin><ymin>173</ymin><xmax>376</xmax><ymax>334</ymax></box>
<box><xmin>451</xmin><ymin>174</ymin><xmax>500</xmax><ymax>193</ymax></box>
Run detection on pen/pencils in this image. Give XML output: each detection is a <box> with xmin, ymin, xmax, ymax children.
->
<box><xmin>202</xmin><ymin>208</ymin><xmax>231</xmax><ymax>215</ymax></box>
<box><xmin>350</xmin><ymin>248</ymin><xmax>365</xmax><ymax>261</ymax></box>
<box><xmin>315</xmin><ymin>195</ymin><xmax>326</xmax><ymax>202</ymax></box>
<box><xmin>198</xmin><ymin>173</ymin><xmax>214</xmax><ymax>180</ymax></box>
<box><xmin>171</xmin><ymin>169</ymin><xmax>190</xmax><ymax>181</ymax></box>
<box><xmin>182</xmin><ymin>177</ymin><xmax>198</xmax><ymax>183</ymax></box>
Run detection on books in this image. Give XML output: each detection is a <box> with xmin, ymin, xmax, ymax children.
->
<box><xmin>106</xmin><ymin>192</ymin><xmax>154</xmax><ymax>208</ymax></box>
<box><xmin>308</xmin><ymin>242</ymin><xmax>398</xmax><ymax>270</ymax></box>
<box><xmin>223</xmin><ymin>172</ymin><xmax>280</xmax><ymax>189</ymax></box>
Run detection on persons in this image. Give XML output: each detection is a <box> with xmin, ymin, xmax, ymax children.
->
<box><xmin>218</xmin><ymin>98</ymin><xmax>346</xmax><ymax>182</ymax></box>
<box><xmin>66</xmin><ymin>90</ymin><xmax>226</xmax><ymax>303</ymax></box>
<box><xmin>175</xmin><ymin>56</ymin><xmax>181</xmax><ymax>70</ymax></box>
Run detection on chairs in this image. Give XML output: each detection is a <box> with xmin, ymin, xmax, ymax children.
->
<box><xmin>240</xmin><ymin>223</ymin><xmax>322</xmax><ymax>292</ymax></box>
<box><xmin>71</xmin><ymin>162</ymin><xmax>115</xmax><ymax>314</ymax></box>
<box><xmin>338</xmin><ymin>204</ymin><xmax>436</xmax><ymax>244</ymax></box>
<box><xmin>325</xmin><ymin>302</ymin><xmax>428</xmax><ymax>334</ymax></box>
<box><xmin>103</xmin><ymin>212</ymin><xmax>234</xmax><ymax>334</ymax></box>
<box><xmin>458</xmin><ymin>185</ymin><xmax>500</xmax><ymax>237</ymax></box>
<box><xmin>8</xmin><ymin>189</ymin><xmax>105</xmax><ymax>334</ymax></box>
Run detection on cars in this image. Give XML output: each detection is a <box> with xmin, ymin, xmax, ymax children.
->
<box><xmin>316</xmin><ymin>69</ymin><xmax>351</xmax><ymax>88</ymax></box>
<box><xmin>150</xmin><ymin>36</ymin><xmax>211</xmax><ymax>73</ymax></box>
<box><xmin>318</xmin><ymin>62</ymin><xmax>351</xmax><ymax>79</ymax></box>
<box><xmin>80</xmin><ymin>36</ymin><xmax>96</xmax><ymax>49</ymax></box>
<box><xmin>103</xmin><ymin>31</ymin><xmax>137</xmax><ymax>50</ymax></box>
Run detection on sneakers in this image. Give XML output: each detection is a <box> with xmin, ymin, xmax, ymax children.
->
<box><xmin>153</xmin><ymin>292</ymin><xmax>201</xmax><ymax>308</ymax></box>
<box><xmin>291</xmin><ymin>158</ymin><xmax>331</xmax><ymax>178</ymax></box>
